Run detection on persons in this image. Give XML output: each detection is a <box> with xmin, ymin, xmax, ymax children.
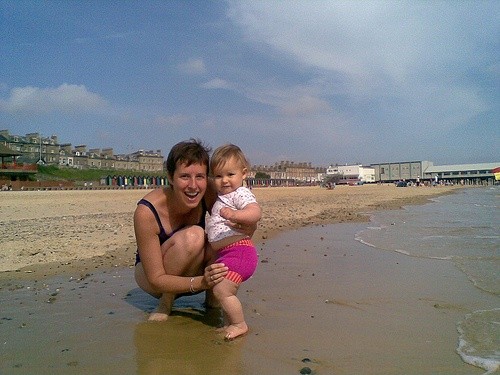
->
<box><xmin>204</xmin><ymin>144</ymin><xmax>262</xmax><ymax>339</ymax></box>
<box><xmin>403</xmin><ymin>176</ymin><xmax>464</xmax><ymax>186</ymax></box>
<box><xmin>36</xmin><ymin>180</ymin><xmax>41</xmax><ymax>191</ymax></box>
<box><xmin>2</xmin><ymin>183</ymin><xmax>13</xmax><ymax>192</ymax></box>
<box><xmin>134</xmin><ymin>138</ymin><xmax>258</xmax><ymax>321</ymax></box>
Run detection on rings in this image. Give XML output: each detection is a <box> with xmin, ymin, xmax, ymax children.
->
<box><xmin>239</xmin><ymin>223</ymin><xmax>242</xmax><ymax>229</ymax></box>
<box><xmin>211</xmin><ymin>275</ymin><xmax>214</xmax><ymax>281</ymax></box>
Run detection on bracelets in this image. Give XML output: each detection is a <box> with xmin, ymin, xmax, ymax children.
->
<box><xmin>190</xmin><ymin>277</ymin><xmax>199</xmax><ymax>293</ymax></box>
